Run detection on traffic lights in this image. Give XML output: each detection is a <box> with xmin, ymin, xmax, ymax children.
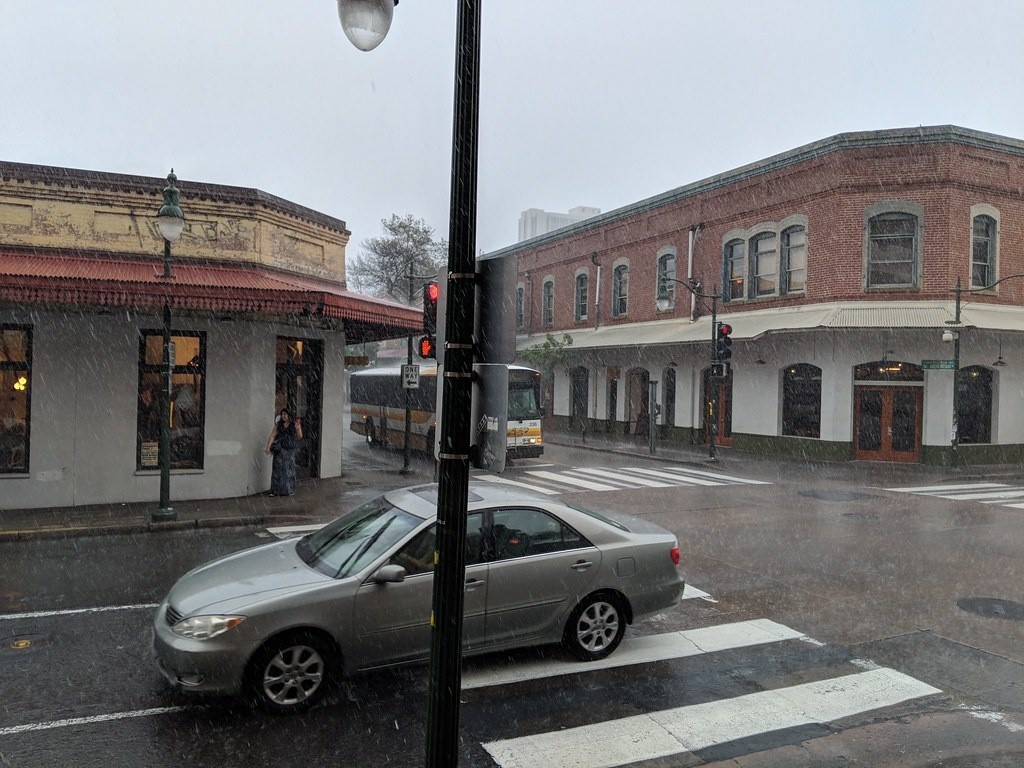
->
<box><xmin>417</xmin><ymin>336</ymin><xmax>436</xmax><ymax>358</ymax></box>
<box><xmin>422</xmin><ymin>280</ymin><xmax>439</xmax><ymax>335</ymax></box>
<box><xmin>716</xmin><ymin>325</ymin><xmax>734</xmax><ymax>360</ymax></box>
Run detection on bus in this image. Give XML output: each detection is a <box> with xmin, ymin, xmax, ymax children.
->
<box><xmin>348</xmin><ymin>362</ymin><xmax>545</xmax><ymax>463</ymax></box>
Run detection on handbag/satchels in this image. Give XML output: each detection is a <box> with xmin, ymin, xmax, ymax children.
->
<box><xmin>270</xmin><ymin>442</ymin><xmax>282</xmax><ymax>455</ymax></box>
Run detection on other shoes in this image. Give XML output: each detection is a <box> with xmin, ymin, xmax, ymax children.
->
<box><xmin>269</xmin><ymin>491</ymin><xmax>277</xmax><ymax>497</ymax></box>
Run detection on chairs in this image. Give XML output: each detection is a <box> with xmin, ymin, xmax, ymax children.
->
<box><xmin>465</xmin><ymin>531</ymin><xmax>485</xmax><ymax>565</ymax></box>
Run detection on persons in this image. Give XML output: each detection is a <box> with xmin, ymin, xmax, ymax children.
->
<box><xmin>266</xmin><ymin>408</ymin><xmax>303</xmax><ymax>495</ymax></box>
<box><xmin>391</xmin><ymin>535</ymin><xmax>435</xmax><ymax>574</ymax></box>
<box><xmin>140</xmin><ymin>388</ymin><xmax>161</xmax><ymax>439</ymax></box>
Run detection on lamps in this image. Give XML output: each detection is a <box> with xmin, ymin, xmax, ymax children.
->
<box><xmin>13</xmin><ymin>374</ymin><xmax>27</xmax><ymax>391</ymax></box>
<box><xmin>752</xmin><ymin>354</ymin><xmax>766</xmax><ymax>365</ymax></box>
<box><xmin>992</xmin><ymin>334</ymin><xmax>1008</xmax><ymax>367</ymax></box>
<box><xmin>667</xmin><ymin>356</ymin><xmax>678</xmax><ymax>367</ymax></box>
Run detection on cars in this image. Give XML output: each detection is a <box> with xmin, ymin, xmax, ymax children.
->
<box><xmin>150</xmin><ymin>481</ymin><xmax>687</xmax><ymax>718</ymax></box>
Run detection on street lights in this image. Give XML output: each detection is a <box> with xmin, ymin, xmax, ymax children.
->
<box><xmin>950</xmin><ymin>274</ymin><xmax>1024</xmax><ymax>468</ymax></box>
<box><xmin>340</xmin><ymin>1</ymin><xmax>485</xmax><ymax>768</ymax></box>
<box><xmin>147</xmin><ymin>169</ymin><xmax>185</xmax><ymax>522</ymax></box>
<box><xmin>657</xmin><ymin>276</ymin><xmax>722</xmax><ymax>464</ymax></box>
